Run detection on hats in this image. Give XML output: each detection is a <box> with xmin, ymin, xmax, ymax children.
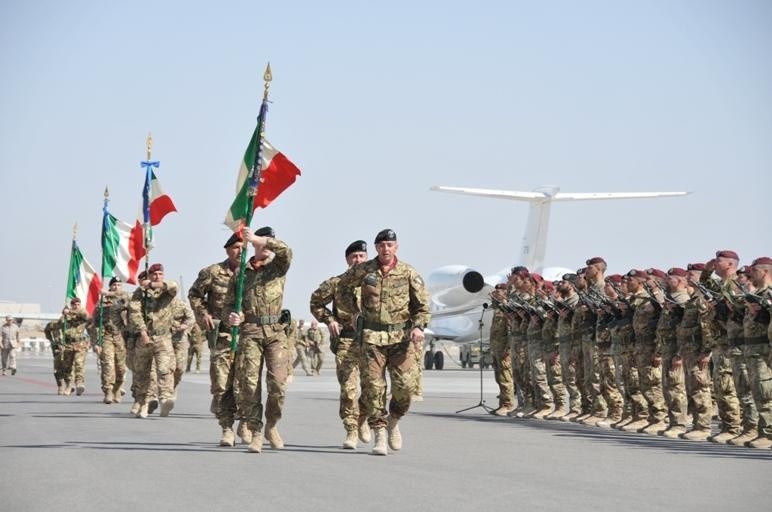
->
<box><xmin>519</xmin><ymin>271</ymin><xmax>533</xmax><ymax>280</ymax></box>
<box><xmin>374</xmin><ymin>229</ymin><xmax>396</xmax><ymax>244</ymax></box>
<box><xmin>627</xmin><ymin>270</ymin><xmax>651</xmax><ymax>279</ymax></box>
<box><xmin>109</xmin><ymin>276</ymin><xmax>121</xmax><ymax>285</ymax></box>
<box><xmin>544</xmin><ymin>281</ymin><xmax>554</xmax><ymax>293</ymax></box>
<box><xmin>668</xmin><ymin>268</ymin><xmax>685</xmax><ymax>277</ymax></box>
<box><xmin>751</xmin><ymin>257</ymin><xmax>772</xmax><ymax>266</ymax></box>
<box><xmin>604</xmin><ymin>275</ymin><xmax>622</xmax><ymax>282</ymax></box>
<box><xmin>252</xmin><ymin>227</ymin><xmax>275</xmax><ymax>247</ymax></box>
<box><xmin>299</xmin><ymin>320</ymin><xmax>304</xmax><ymax>324</ymax></box>
<box><xmin>736</xmin><ymin>266</ymin><xmax>751</xmax><ymax>276</ymax></box>
<box><xmin>6</xmin><ymin>315</ymin><xmax>13</xmax><ymax>321</ymax></box>
<box><xmin>224</xmin><ymin>233</ymin><xmax>243</xmax><ymax>248</ymax></box>
<box><xmin>647</xmin><ymin>268</ymin><xmax>665</xmax><ymax>278</ymax></box>
<box><xmin>495</xmin><ymin>284</ymin><xmax>506</xmax><ymax>289</ymax></box>
<box><xmin>577</xmin><ymin>267</ymin><xmax>587</xmax><ymax>274</ymax></box>
<box><xmin>346</xmin><ymin>241</ymin><xmax>366</xmax><ymax>257</ymax></box>
<box><xmin>688</xmin><ymin>264</ymin><xmax>705</xmax><ymax>270</ymax></box>
<box><xmin>71</xmin><ymin>298</ymin><xmax>80</xmax><ymax>304</ymax></box>
<box><xmin>563</xmin><ymin>274</ymin><xmax>576</xmax><ymax>283</ymax></box>
<box><xmin>148</xmin><ymin>264</ymin><xmax>163</xmax><ymax>273</ymax></box>
<box><xmin>716</xmin><ymin>251</ymin><xmax>738</xmax><ymax>258</ymax></box>
<box><xmin>138</xmin><ymin>271</ymin><xmax>146</xmax><ymax>279</ymax></box>
<box><xmin>586</xmin><ymin>257</ymin><xmax>602</xmax><ymax>265</ymax></box>
<box><xmin>532</xmin><ymin>273</ymin><xmax>544</xmax><ymax>285</ymax></box>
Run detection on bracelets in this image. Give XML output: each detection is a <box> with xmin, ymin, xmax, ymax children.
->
<box><xmin>414</xmin><ymin>325</ymin><xmax>425</xmax><ymax>332</ymax></box>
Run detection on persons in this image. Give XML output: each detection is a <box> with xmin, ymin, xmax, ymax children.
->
<box><xmin>619</xmin><ymin>268</ymin><xmax>670</xmax><ymax>434</ymax></box>
<box><xmin>110</xmin><ymin>271</ymin><xmax>160</xmax><ymax>414</ymax></box>
<box><xmin>580</xmin><ymin>256</ymin><xmax>608</xmax><ymax>426</ymax></box>
<box><xmin>540</xmin><ymin>281</ymin><xmax>571</xmax><ymax>421</ymax></box>
<box><xmin>291</xmin><ymin>319</ymin><xmax>313</xmax><ymax>376</ymax></box>
<box><xmin>46</xmin><ymin>306</ymin><xmax>76</xmax><ymax>395</ymax></box>
<box><xmin>520</xmin><ymin>273</ymin><xmax>577</xmax><ymax>419</ymax></box>
<box><xmin>170</xmin><ymin>299</ymin><xmax>196</xmax><ymax>389</ymax></box>
<box><xmin>220</xmin><ymin>226</ymin><xmax>289</xmax><ymax>453</ymax></box>
<box><xmin>620</xmin><ymin>275</ymin><xmax>633</xmax><ymax>304</ymax></box>
<box><xmin>646</xmin><ymin>268</ymin><xmax>688</xmax><ymax>439</ymax></box>
<box><xmin>543</xmin><ymin>281</ymin><xmax>564</xmax><ymax>303</ymax></box>
<box><xmin>489</xmin><ymin>283</ymin><xmax>518</xmax><ymax>416</ymax></box>
<box><xmin>55</xmin><ymin>298</ymin><xmax>94</xmax><ymax>398</ymax></box>
<box><xmin>679</xmin><ymin>263</ymin><xmax>717</xmax><ymax>441</ymax></box>
<box><xmin>185</xmin><ymin>321</ymin><xmax>207</xmax><ymax>373</ymax></box>
<box><xmin>86</xmin><ymin>276</ymin><xmax>131</xmax><ymax>404</ymax></box>
<box><xmin>574</xmin><ymin>267</ymin><xmax>593</xmax><ymax>422</ymax></box>
<box><xmin>306</xmin><ymin>320</ymin><xmax>326</xmax><ymax>375</ymax></box>
<box><xmin>662</xmin><ymin>267</ymin><xmax>689</xmax><ymax>440</ymax></box>
<box><xmin>736</xmin><ymin>265</ymin><xmax>758</xmax><ymax>295</ymax></box>
<box><xmin>337</xmin><ymin>229</ymin><xmax>432</xmax><ymax>455</ymax></box>
<box><xmin>310</xmin><ymin>239</ymin><xmax>372</xmax><ymax>450</ymax></box>
<box><xmin>506</xmin><ymin>266</ymin><xmax>538</xmax><ymax>419</ymax></box>
<box><xmin>699</xmin><ymin>250</ymin><xmax>744</xmax><ymax>445</ymax></box>
<box><xmin>596</xmin><ymin>274</ymin><xmax>633</xmax><ymax>428</ymax></box>
<box><xmin>0</xmin><ymin>314</ymin><xmax>22</xmax><ymax>376</ymax></box>
<box><xmin>727</xmin><ymin>256</ymin><xmax>772</xmax><ymax>450</ymax></box>
<box><xmin>187</xmin><ymin>231</ymin><xmax>253</xmax><ymax>447</ymax></box>
<box><xmin>127</xmin><ymin>263</ymin><xmax>179</xmax><ymax>419</ymax></box>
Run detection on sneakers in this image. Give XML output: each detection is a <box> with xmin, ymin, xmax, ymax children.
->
<box><xmin>160</xmin><ymin>399</ymin><xmax>175</xmax><ymax>417</ymax></box>
<box><xmin>748</xmin><ymin>437</ymin><xmax>771</xmax><ymax>448</ymax></box>
<box><xmin>611</xmin><ymin>416</ymin><xmax>633</xmax><ymax>429</ymax></box>
<box><xmin>2</xmin><ymin>370</ymin><xmax>6</xmax><ymax>375</ymax></box>
<box><xmin>306</xmin><ymin>372</ymin><xmax>314</xmax><ymax>376</ymax></box>
<box><xmin>728</xmin><ymin>430</ymin><xmax>758</xmax><ymax>445</ymax></box>
<box><xmin>495</xmin><ymin>405</ymin><xmax>513</xmax><ymax>416</ymax></box>
<box><xmin>621</xmin><ymin>419</ymin><xmax>650</xmax><ymax>432</ymax></box>
<box><xmin>543</xmin><ymin>410</ymin><xmax>570</xmax><ymax>420</ymax></box>
<box><xmin>642</xmin><ymin>420</ymin><xmax>669</xmax><ymax>434</ymax></box>
<box><xmin>148</xmin><ymin>398</ymin><xmax>159</xmax><ymax>414</ymax></box>
<box><xmin>531</xmin><ymin>409</ymin><xmax>551</xmax><ymax>419</ymax></box>
<box><xmin>136</xmin><ymin>404</ymin><xmax>148</xmax><ymax>418</ymax></box>
<box><xmin>523</xmin><ymin>409</ymin><xmax>538</xmax><ymax>418</ymax></box>
<box><xmin>581</xmin><ymin>415</ymin><xmax>606</xmax><ymax>426</ymax></box>
<box><xmin>64</xmin><ymin>381</ymin><xmax>72</xmax><ymax>395</ymax></box>
<box><xmin>12</xmin><ymin>369</ymin><xmax>17</xmax><ymax>375</ymax></box>
<box><xmin>570</xmin><ymin>413</ymin><xmax>591</xmax><ymax>422</ymax></box>
<box><xmin>663</xmin><ymin>425</ymin><xmax>687</xmax><ymax>438</ymax></box>
<box><xmin>596</xmin><ymin>418</ymin><xmax>620</xmax><ymax>428</ymax></box>
<box><xmin>712</xmin><ymin>432</ymin><xmax>742</xmax><ymax>444</ymax></box>
<box><xmin>560</xmin><ymin>412</ymin><xmax>578</xmax><ymax>421</ymax></box>
<box><xmin>517</xmin><ymin>407</ymin><xmax>535</xmax><ymax>417</ymax></box>
<box><xmin>76</xmin><ymin>381</ymin><xmax>85</xmax><ymax>395</ymax></box>
<box><xmin>131</xmin><ymin>402</ymin><xmax>140</xmax><ymax>413</ymax></box>
<box><xmin>58</xmin><ymin>379</ymin><xmax>64</xmax><ymax>395</ymax></box>
<box><xmin>682</xmin><ymin>427</ymin><xmax>712</xmax><ymax>440</ymax></box>
<box><xmin>507</xmin><ymin>407</ymin><xmax>524</xmax><ymax>416</ymax></box>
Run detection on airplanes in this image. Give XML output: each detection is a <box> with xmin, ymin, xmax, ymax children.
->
<box><xmin>411</xmin><ymin>186</ymin><xmax>690</xmax><ymax>371</ymax></box>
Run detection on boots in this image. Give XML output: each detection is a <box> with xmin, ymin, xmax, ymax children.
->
<box><xmin>237</xmin><ymin>419</ymin><xmax>252</xmax><ymax>445</ymax></box>
<box><xmin>112</xmin><ymin>381</ymin><xmax>123</xmax><ymax>403</ymax></box>
<box><xmin>359</xmin><ymin>413</ymin><xmax>371</xmax><ymax>443</ymax></box>
<box><xmin>384</xmin><ymin>414</ymin><xmax>401</xmax><ymax>451</ymax></box>
<box><xmin>220</xmin><ymin>426</ymin><xmax>235</xmax><ymax>446</ymax></box>
<box><xmin>372</xmin><ymin>427</ymin><xmax>387</xmax><ymax>454</ymax></box>
<box><xmin>343</xmin><ymin>429</ymin><xmax>358</xmax><ymax>449</ymax></box>
<box><xmin>265</xmin><ymin>420</ymin><xmax>284</xmax><ymax>449</ymax></box>
<box><xmin>104</xmin><ymin>388</ymin><xmax>113</xmax><ymax>404</ymax></box>
<box><xmin>248</xmin><ymin>429</ymin><xmax>263</xmax><ymax>453</ymax></box>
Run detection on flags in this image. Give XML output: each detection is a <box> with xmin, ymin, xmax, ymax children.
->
<box><xmin>63</xmin><ymin>241</ymin><xmax>104</xmax><ymax>319</ymax></box>
<box><xmin>134</xmin><ymin>159</ymin><xmax>178</xmax><ymax>262</ymax></box>
<box><xmin>224</xmin><ymin>100</ymin><xmax>302</xmax><ymax>235</ymax></box>
<box><xmin>100</xmin><ymin>209</ymin><xmax>138</xmax><ymax>286</ymax></box>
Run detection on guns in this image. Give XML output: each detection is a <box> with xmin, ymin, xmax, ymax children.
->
<box><xmin>731</xmin><ymin>280</ymin><xmax>769</xmax><ymax>323</ymax></box>
<box><xmin>571</xmin><ymin>285</ymin><xmax>597</xmax><ymax>320</ymax></box>
<box><xmin>487</xmin><ymin>294</ymin><xmax>505</xmax><ymax>314</ymax></box>
<box><xmin>712</xmin><ymin>278</ymin><xmax>745</xmax><ymax>320</ymax></box>
<box><xmin>538</xmin><ymin>289</ymin><xmax>574</xmax><ymax>320</ymax></box>
<box><xmin>488</xmin><ymin>293</ymin><xmax>519</xmax><ymax>321</ymax></box>
<box><xmin>590</xmin><ymin>289</ymin><xmax>623</xmax><ymax>321</ymax></box>
<box><xmin>653</xmin><ymin>280</ymin><xmax>684</xmax><ymax>319</ymax></box>
<box><xmin>513</xmin><ymin>293</ymin><xmax>545</xmax><ymax>320</ymax></box>
<box><xmin>582</xmin><ymin>289</ymin><xmax>611</xmax><ymax>320</ymax></box>
<box><xmin>642</xmin><ymin>280</ymin><xmax>662</xmax><ymax>320</ymax></box>
<box><xmin>608</xmin><ymin>279</ymin><xmax>634</xmax><ymax>320</ymax></box>
<box><xmin>525</xmin><ymin>289</ymin><xmax>560</xmax><ymax>320</ymax></box>
<box><xmin>690</xmin><ymin>279</ymin><xmax>728</xmax><ymax>321</ymax></box>
<box><xmin>498</xmin><ymin>293</ymin><xmax>530</xmax><ymax>320</ymax></box>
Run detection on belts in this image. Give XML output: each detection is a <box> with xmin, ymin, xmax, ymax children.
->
<box><xmin>528</xmin><ymin>336</ymin><xmax>542</xmax><ymax>340</ymax></box>
<box><xmin>148</xmin><ymin>329</ymin><xmax>167</xmax><ymax>336</ymax></box>
<box><xmin>727</xmin><ymin>338</ymin><xmax>744</xmax><ymax>345</ymax></box>
<box><xmin>105</xmin><ymin>330</ymin><xmax>121</xmax><ymax>335</ymax></box>
<box><xmin>570</xmin><ymin>333</ymin><xmax>579</xmax><ymax>340</ymax></box>
<box><xmin>677</xmin><ymin>333</ymin><xmax>701</xmax><ymax>342</ymax></box>
<box><xmin>659</xmin><ymin>336</ymin><xmax>674</xmax><ymax>344</ymax></box>
<box><xmin>519</xmin><ymin>335</ymin><xmax>527</xmax><ymax>340</ymax></box>
<box><xmin>512</xmin><ymin>332</ymin><xmax>522</xmax><ymax>336</ymax></box>
<box><xmin>340</xmin><ymin>331</ymin><xmax>357</xmax><ymax>338</ymax></box>
<box><xmin>128</xmin><ymin>333</ymin><xmax>137</xmax><ymax>338</ymax></box>
<box><xmin>559</xmin><ymin>335</ymin><xmax>572</xmax><ymax>343</ymax></box>
<box><xmin>244</xmin><ymin>314</ymin><xmax>278</xmax><ymax>324</ymax></box>
<box><xmin>543</xmin><ymin>340</ymin><xmax>552</xmax><ymax>344</ymax></box>
<box><xmin>743</xmin><ymin>335</ymin><xmax>768</xmax><ymax>344</ymax></box>
<box><xmin>595</xmin><ymin>341</ymin><xmax>609</xmax><ymax>348</ymax></box>
<box><xmin>67</xmin><ymin>337</ymin><xmax>86</xmax><ymax>342</ymax></box>
<box><xmin>363</xmin><ymin>320</ymin><xmax>411</xmax><ymax>332</ymax></box>
<box><xmin>4</xmin><ymin>338</ymin><xmax>16</xmax><ymax>340</ymax></box>
<box><xmin>611</xmin><ymin>335</ymin><xmax>632</xmax><ymax>345</ymax></box>
<box><xmin>220</xmin><ymin>326</ymin><xmax>240</xmax><ymax>334</ymax></box>
<box><xmin>634</xmin><ymin>336</ymin><xmax>655</xmax><ymax>344</ymax></box>
<box><xmin>580</xmin><ymin>328</ymin><xmax>592</xmax><ymax>336</ymax></box>
